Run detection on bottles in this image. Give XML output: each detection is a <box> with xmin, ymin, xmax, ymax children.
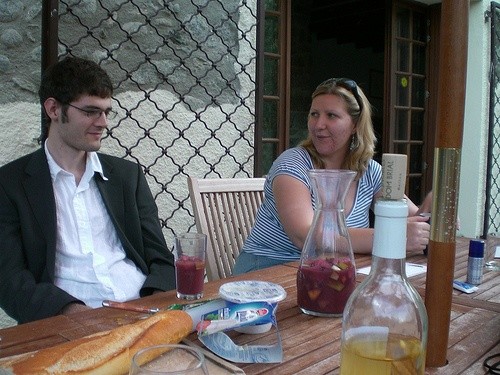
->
<box><xmin>295</xmin><ymin>167</ymin><xmax>358</xmax><ymax>317</ymax></box>
<box><xmin>338</xmin><ymin>154</ymin><xmax>428</xmax><ymax>375</ymax></box>
<box><xmin>466</xmin><ymin>239</ymin><xmax>485</xmax><ymax>285</ymax></box>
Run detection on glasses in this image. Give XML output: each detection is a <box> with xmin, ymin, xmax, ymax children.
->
<box><xmin>56</xmin><ymin>99</ymin><xmax>118</xmax><ymax>120</ymax></box>
<box><xmin>320</xmin><ymin>78</ymin><xmax>362</xmax><ymax>115</ymax></box>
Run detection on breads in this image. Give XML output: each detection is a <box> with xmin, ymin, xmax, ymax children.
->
<box><xmin>0</xmin><ymin>309</ymin><xmax>194</xmax><ymax>375</ymax></box>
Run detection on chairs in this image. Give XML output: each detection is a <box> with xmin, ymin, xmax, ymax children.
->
<box><xmin>187</xmin><ymin>177</ymin><xmax>266</xmax><ymax>281</ymax></box>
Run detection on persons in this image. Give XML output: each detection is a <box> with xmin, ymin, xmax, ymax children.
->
<box><xmin>232</xmin><ymin>77</ymin><xmax>460</xmax><ymax>279</ymax></box>
<box><xmin>0</xmin><ymin>55</ymin><xmax>175</xmax><ymax>325</ymax></box>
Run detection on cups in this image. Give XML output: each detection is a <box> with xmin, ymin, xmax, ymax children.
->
<box><xmin>129</xmin><ymin>343</ymin><xmax>210</xmax><ymax>375</ymax></box>
<box><xmin>219</xmin><ymin>280</ymin><xmax>287</xmax><ymax>334</ymax></box>
<box><xmin>174</xmin><ymin>232</ymin><xmax>207</xmax><ymax>300</ymax></box>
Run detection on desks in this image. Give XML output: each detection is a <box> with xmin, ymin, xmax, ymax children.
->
<box><xmin>0</xmin><ymin>236</ymin><xmax>500</xmax><ymax>375</ymax></box>
